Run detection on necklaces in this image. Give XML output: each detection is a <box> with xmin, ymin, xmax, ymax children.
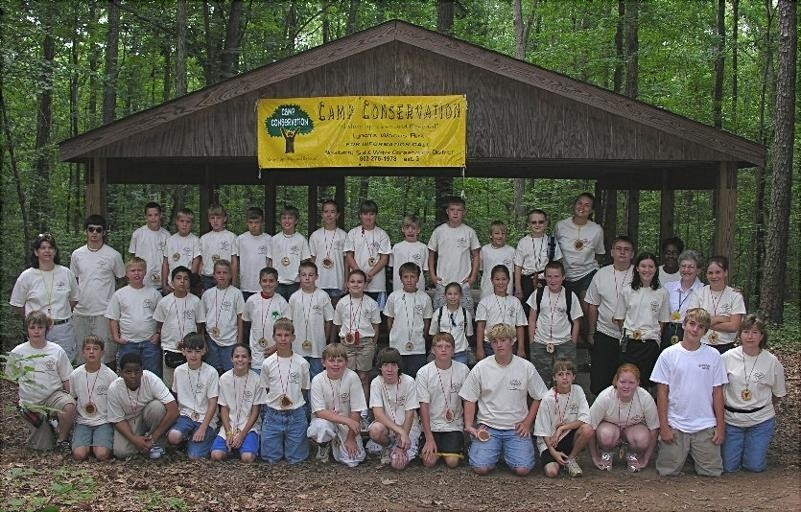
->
<box><xmin>87</xmin><ymin>241</ymin><xmax>104</xmax><ymax>251</ymax></box>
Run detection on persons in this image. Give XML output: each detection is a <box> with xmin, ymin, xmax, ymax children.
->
<box><xmin>200</xmin><ymin>203</ymin><xmax>237</xmax><ymax>296</ymax></box>
<box><xmin>271</xmin><ymin>206</ymin><xmax>312</xmax><ymax>302</ymax></box>
<box><xmin>458</xmin><ymin>323</ymin><xmax>549</xmax><ymax>476</ymax></box>
<box><xmin>660</xmin><ymin>249</ymin><xmax>701</xmax><ymax>355</ymax></box>
<box><xmin>68</xmin><ymin>336</ymin><xmax>117</xmax><ymax>460</ymax></box>
<box><xmin>210</xmin><ymin>343</ymin><xmax>261</xmax><ymax>463</ymax></box>
<box><xmin>168</xmin><ymin>331</ymin><xmax>219</xmax><ymax>460</ymax></box>
<box><xmin>288</xmin><ymin>262</ymin><xmax>333</xmax><ymax>380</ymax></box>
<box><xmin>331</xmin><ymin>267</ymin><xmax>382</xmax><ymax>397</ymax></box>
<box><xmin>9</xmin><ymin>234</ymin><xmax>78</xmax><ymax>363</ymax></box>
<box><xmin>128</xmin><ymin>202</ymin><xmax>171</xmax><ymax>296</ymax></box>
<box><xmin>479</xmin><ymin>221</ymin><xmax>516</xmax><ymax>299</ymax></box>
<box><xmin>427</xmin><ymin>196</ymin><xmax>481</xmax><ymax>319</ymax></box>
<box><xmin>235</xmin><ymin>207</ymin><xmax>272</xmax><ymax>302</ymax></box>
<box><xmin>584</xmin><ymin>235</ymin><xmax>635</xmax><ymax>396</ymax></box>
<box><xmin>70</xmin><ymin>214</ymin><xmax>125</xmax><ymax>366</ymax></box>
<box><xmin>533</xmin><ymin>356</ymin><xmax>592</xmax><ymax>480</ymax></box>
<box><xmin>553</xmin><ymin>193</ymin><xmax>605</xmax><ymax>311</ymax></box>
<box><xmin>650</xmin><ymin>309</ymin><xmax>728</xmax><ymax>479</ymax></box>
<box><xmin>686</xmin><ymin>256</ymin><xmax>747</xmax><ymax>353</ymax></box>
<box><xmin>389</xmin><ymin>216</ymin><xmax>429</xmax><ymax>292</ymax></box>
<box><xmin>105</xmin><ymin>257</ymin><xmax>163</xmax><ymax>379</ymax></box>
<box><xmin>654</xmin><ymin>236</ymin><xmax>687</xmax><ymax>287</ymax></box>
<box><xmin>242</xmin><ymin>265</ymin><xmax>292</xmax><ymax>376</ymax></box>
<box><xmin>307</xmin><ymin>342</ymin><xmax>368</xmax><ymax>468</ymax></box>
<box><xmin>200</xmin><ymin>259</ymin><xmax>245</xmax><ymax>376</ymax></box>
<box><xmin>526</xmin><ymin>262</ymin><xmax>584</xmax><ymax>388</ymax></box>
<box><xmin>107</xmin><ymin>353</ymin><xmax>178</xmax><ymax>458</ymax></box>
<box><xmin>474</xmin><ymin>264</ymin><xmax>528</xmax><ymax>362</ymax></box>
<box><xmin>429</xmin><ymin>282</ymin><xmax>473</xmax><ymax>367</ymax></box>
<box><xmin>720</xmin><ymin>315</ymin><xmax>787</xmax><ymax>473</ymax></box>
<box><xmin>613</xmin><ymin>253</ymin><xmax>670</xmax><ymax>387</ymax></box>
<box><xmin>590</xmin><ymin>364</ymin><xmax>660</xmax><ymax>472</ymax></box>
<box><xmin>415</xmin><ymin>333</ymin><xmax>470</xmax><ymax>469</ymax></box>
<box><xmin>308</xmin><ymin>200</ymin><xmax>347</xmax><ymax>301</ymax></box>
<box><xmin>382</xmin><ymin>262</ymin><xmax>433</xmax><ymax>378</ymax></box>
<box><xmin>515</xmin><ymin>209</ymin><xmax>564</xmax><ymax>318</ymax></box>
<box><xmin>344</xmin><ymin>200</ymin><xmax>391</xmax><ymax>301</ymax></box>
<box><xmin>161</xmin><ymin>207</ymin><xmax>202</xmax><ymax>295</ymax></box>
<box><xmin>4</xmin><ymin>309</ymin><xmax>73</xmax><ymax>455</ymax></box>
<box><xmin>368</xmin><ymin>348</ymin><xmax>421</xmax><ymax>470</ymax></box>
<box><xmin>260</xmin><ymin>317</ymin><xmax>311</xmax><ymax>463</ymax></box>
<box><xmin>152</xmin><ymin>266</ymin><xmax>205</xmax><ymax>391</ymax></box>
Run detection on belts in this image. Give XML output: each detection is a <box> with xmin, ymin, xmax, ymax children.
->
<box><xmin>53</xmin><ymin>319</ymin><xmax>68</xmax><ymax>325</ymax></box>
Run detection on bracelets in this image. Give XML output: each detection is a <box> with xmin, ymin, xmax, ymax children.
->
<box><xmin>225</xmin><ymin>432</ymin><xmax>232</xmax><ymax>436</ymax></box>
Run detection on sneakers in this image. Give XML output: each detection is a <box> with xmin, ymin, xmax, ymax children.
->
<box><xmin>315</xmin><ymin>443</ymin><xmax>331</xmax><ymax>463</ymax></box>
<box><xmin>381</xmin><ymin>444</ymin><xmax>393</xmax><ymax>465</ymax></box>
<box><xmin>57</xmin><ymin>440</ymin><xmax>72</xmax><ymax>458</ymax></box>
<box><xmin>150</xmin><ymin>445</ymin><xmax>163</xmax><ymax>458</ymax></box>
<box><xmin>564</xmin><ymin>458</ymin><xmax>583</xmax><ymax>477</ymax></box>
<box><xmin>623</xmin><ymin>453</ymin><xmax>640</xmax><ymax>473</ymax></box>
<box><xmin>600</xmin><ymin>452</ymin><xmax>613</xmax><ymax>472</ymax></box>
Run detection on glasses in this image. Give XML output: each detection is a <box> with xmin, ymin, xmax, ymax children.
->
<box><xmin>87</xmin><ymin>227</ymin><xmax>102</xmax><ymax>233</ymax></box>
<box><xmin>532</xmin><ymin>221</ymin><xmax>544</xmax><ymax>223</ymax></box>
<box><xmin>665</xmin><ymin>250</ymin><xmax>680</xmax><ymax>256</ymax></box>
<box><xmin>38</xmin><ymin>234</ymin><xmax>51</xmax><ymax>240</ymax></box>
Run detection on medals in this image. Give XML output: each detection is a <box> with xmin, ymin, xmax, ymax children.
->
<box><xmin>445</xmin><ymin>408</ymin><xmax>455</xmax><ymax>423</ymax></box>
<box><xmin>671</xmin><ymin>312</ymin><xmax>680</xmax><ymax>321</ymax></box>
<box><xmin>302</xmin><ymin>340</ymin><xmax>312</xmax><ymax>350</ymax></box>
<box><xmin>546</xmin><ymin>342</ymin><xmax>554</xmax><ymax>354</ymax></box>
<box><xmin>740</xmin><ymin>389</ymin><xmax>752</xmax><ymax>401</ymax></box>
<box><xmin>344</xmin><ymin>332</ymin><xmax>355</xmax><ymax>345</ymax></box>
<box><xmin>708</xmin><ymin>333</ymin><xmax>718</xmax><ymax>344</ymax></box>
<box><xmin>47</xmin><ymin>317</ymin><xmax>54</xmax><ymax>328</ymax></box>
<box><xmin>368</xmin><ymin>257</ymin><xmax>376</xmax><ymax>266</ymax></box>
<box><xmin>574</xmin><ymin>240</ymin><xmax>585</xmax><ymax>251</ymax></box>
<box><xmin>281</xmin><ymin>397</ymin><xmax>291</xmax><ymax>406</ymax></box>
<box><xmin>211</xmin><ymin>327</ymin><xmax>220</xmax><ymax>337</ymax></box>
<box><xmin>477</xmin><ymin>429</ymin><xmax>490</xmax><ymax>442</ymax></box>
<box><xmin>633</xmin><ymin>330</ymin><xmax>641</xmax><ymax>340</ymax></box>
<box><xmin>619</xmin><ymin>448</ymin><xmax>624</xmax><ymax>459</ymax></box>
<box><xmin>281</xmin><ymin>257</ymin><xmax>290</xmax><ymax>266</ymax></box>
<box><xmin>233</xmin><ymin>428</ymin><xmax>241</xmax><ymax>436</ymax></box>
<box><xmin>406</xmin><ymin>342</ymin><xmax>414</xmax><ymax>351</ymax></box>
<box><xmin>259</xmin><ymin>337</ymin><xmax>268</xmax><ymax>348</ymax></box>
<box><xmin>172</xmin><ymin>252</ymin><xmax>180</xmax><ymax>262</ymax></box>
<box><xmin>322</xmin><ymin>257</ymin><xmax>333</xmax><ymax>268</ymax></box>
<box><xmin>84</xmin><ymin>402</ymin><xmax>96</xmax><ymax>416</ymax></box>
<box><xmin>190</xmin><ymin>411</ymin><xmax>200</xmax><ymax>422</ymax></box>
